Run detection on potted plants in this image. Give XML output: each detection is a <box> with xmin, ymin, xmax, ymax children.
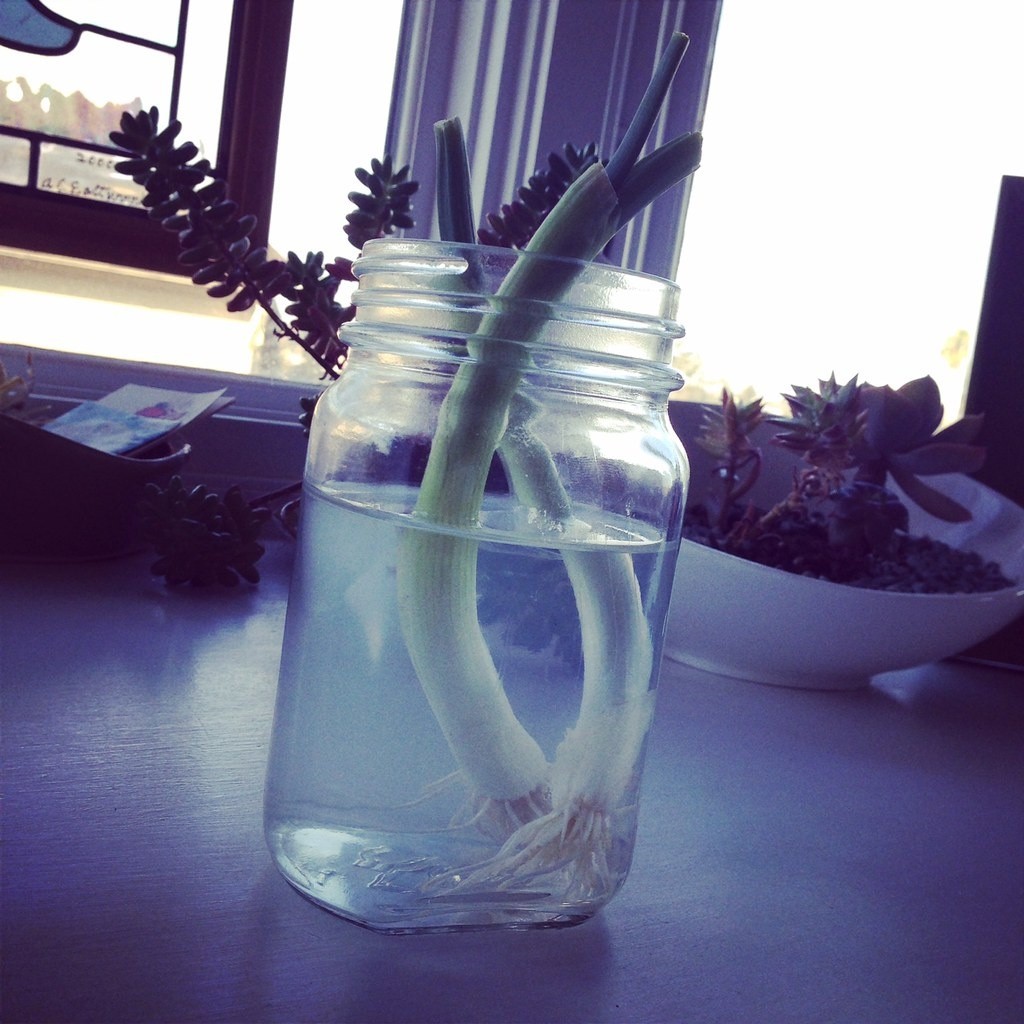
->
<box><xmin>261</xmin><ymin>33</ymin><xmax>702</xmax><ymax>934</ymax></box>
<box><xmin>662</xmin><ymin>378</ymin><xmax>1024</xmax><ymax>691</ymax></box>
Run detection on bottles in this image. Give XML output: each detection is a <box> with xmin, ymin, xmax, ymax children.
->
<box><xmin>264</xmin><ymin>238</ymin><xmax>692</xmax><ymax>933</ymax></box>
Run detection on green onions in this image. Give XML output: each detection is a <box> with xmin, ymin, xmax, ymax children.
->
<box><xmin>396</xmin><ymin>30</ymin><xmax>701</xmax><ymax>836</ymax></box>
<box><xmin>433</xmin><ymin>119</ymin><xmax>654</xmax><ymax>905</ymax></box>
